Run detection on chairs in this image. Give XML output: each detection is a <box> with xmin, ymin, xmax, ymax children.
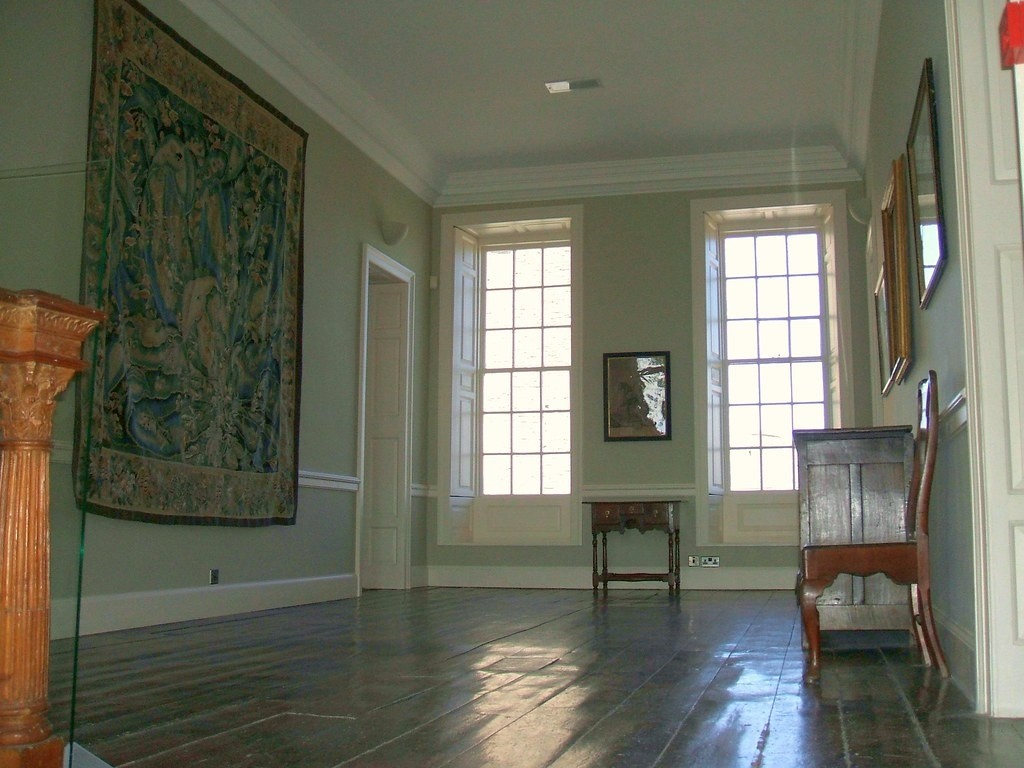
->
<box><xmin>798</xmin><ymin>370</ymin><xmax>951</xmax><ymax>685</ymax></box>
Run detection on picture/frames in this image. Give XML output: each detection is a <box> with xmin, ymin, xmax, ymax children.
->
<box><xmin>603</xmin><ymin>350</ymin><xmax>672</xmax><ymax>444</ymax></box>
<box><xmin>906</xmin><ymin>57</ymin><xmax>947</xmax><ymax>311</ymax></box>
<box><xmin>873</xmin><ymin>262</ymin><xmax>901</xmax><ymax>398</ymax></box>
<box><xmin>880</xmin><ymin>153</ymin><xmax>913</xmax><ymax>386</ymax></box>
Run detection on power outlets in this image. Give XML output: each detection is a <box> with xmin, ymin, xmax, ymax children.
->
<box><xmin>702</xmin><ymin>556</ymin><xmax>720</xmax><ymax>568</ymax></box>
<box><xmin>210</xmin><ymin>569</ymin><xmax>219</xmax><ymax>585</ymax></box>
<box><xmin>688</xmin><ymin>556</ymin><xmax>700</xmax><ymax>567</ymax></box>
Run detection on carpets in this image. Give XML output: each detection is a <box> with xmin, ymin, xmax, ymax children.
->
<box><xmin>72</xmin><ymin>0</ymin><xmax>311</xmax><ymax>527</ymax></box>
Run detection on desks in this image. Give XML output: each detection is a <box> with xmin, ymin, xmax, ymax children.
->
<box><xmin>582</xmin><ymin>500</ymin><xmax>682</xmax><ymax>597</ymax></box>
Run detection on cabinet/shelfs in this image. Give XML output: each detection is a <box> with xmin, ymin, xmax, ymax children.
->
<box><xmin>792</xmin><ymin>425</ymin><xmax>924</xmax><ymax>650</ymax></box>
